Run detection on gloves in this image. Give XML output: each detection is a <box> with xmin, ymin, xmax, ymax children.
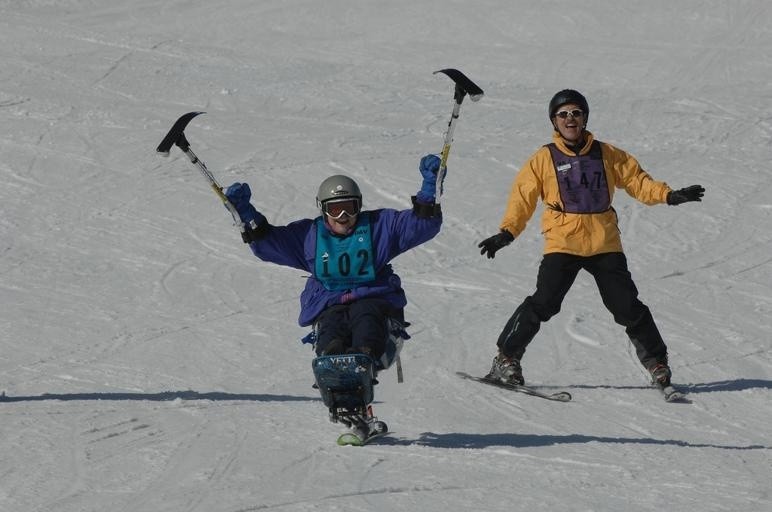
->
<box><xmin>478</xmin><ymin>229</ymin><xmax>515</xmax><ymax>259</ymax></box>
<box><xmin>223</xmin><ymin>182</ymin><xmax>258</xmax><ymax>227</ymax></box>
<box><xmin>666</xmin><ymin>184</ymin><xmax>706</xmax><ymax>207</ymax></box>
<box><xmin>418</xmin><ymin>153</ymin><xmax>447</xmax><ymax>203</ymax></box>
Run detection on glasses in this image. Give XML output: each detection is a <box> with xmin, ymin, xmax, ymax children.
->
<box><xmin>553</xmin><ymin>109</ymin><xmax>582</xmax><ymax>118</ymax></box>
<box><xmin>320</xmin><ymin>198</ymin><xmax>361</xmax><ymax>219</ymax></box>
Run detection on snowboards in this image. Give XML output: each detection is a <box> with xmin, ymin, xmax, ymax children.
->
<box><xmin>312</xmin><ymin>354</ymin><xmax>375</xmax><ymax>406</ymax></box>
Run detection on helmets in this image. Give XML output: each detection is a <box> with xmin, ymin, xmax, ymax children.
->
<box><xmin>548</xmin><ymin>88</ymin><xmax>590</xmax><ymax>138</ymax></box>
<box><xmin>316</xmin><ymin>173</ymin><xmax>363</xmax><ymax>220</ymax></box>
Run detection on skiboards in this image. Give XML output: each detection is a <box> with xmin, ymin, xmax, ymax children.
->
<box><xmin>454</xmin><ymin>370</ymin><xmax>687</xmax><ymax>402</ymax></box>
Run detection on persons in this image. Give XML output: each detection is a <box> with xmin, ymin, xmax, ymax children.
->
<box><xmin>477</xmin><ymin>89</ymin><xmax>705</xmax><ymax>383</ymax></box>
<box><xmin>225</xmin><ymin>153</ymin><xmax>447</xmax><ymax>387</ymax></box>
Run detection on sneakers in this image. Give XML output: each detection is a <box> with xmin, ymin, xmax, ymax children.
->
<box><xmin>645</xmin><ymin>355</ymin><xmax>672</xmax><ymax>383</ymax></box>
<box><xmin>352</xmin><ymin>404</ymin><xmax>378</xmax><ymax>444</ymax></box>
<box><xmin>491</xmin><ymin>353</ymin><xmax>525</xmax><ymax>387</ymax></box>
